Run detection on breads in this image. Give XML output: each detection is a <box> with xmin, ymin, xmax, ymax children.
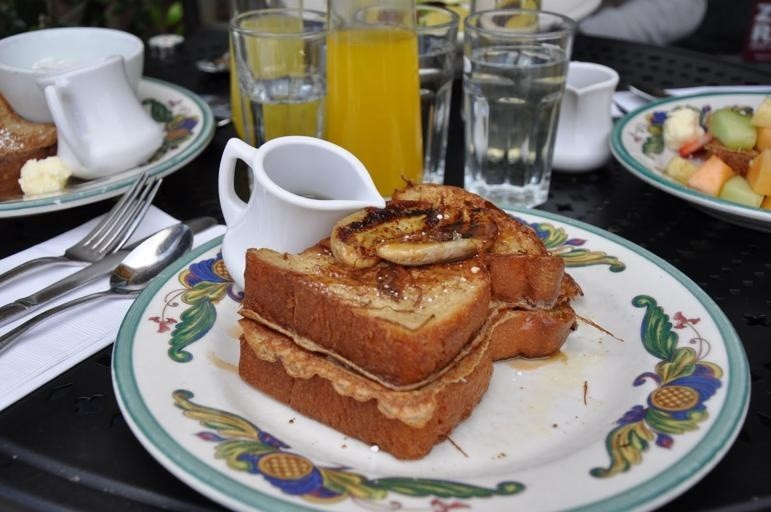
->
<box><xmin>0</xmin><ymin>91</ymin><xmax>60</xmax><ymax>205</ymax></box>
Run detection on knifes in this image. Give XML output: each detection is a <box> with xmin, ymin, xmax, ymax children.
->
<box><xmin>1</xmin><ymin>216</ymin><xmax>217</xmax><ymax>328</ymax></box>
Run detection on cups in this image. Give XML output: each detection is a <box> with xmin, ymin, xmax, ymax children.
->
<box><xmin>352</xmin><ymin>5</ymin><xmax>460</xmax><ymax>184</ymax></box>
<box><xmin>551</xmin><ymin>59</ymin><xmax>618</xmax><ymax>174</ymax></box>
<box><xmin>462</xmin><ymin>10</ymin><xmax>577</xmax><ymax>207</ymax></box>
<box><xmin>229</xmin><ymin>8</ymin><xmax>343</xmax><ymax>145</ymax></box>
<box><xmin>35</xmin><ymin>54</ymin><xmax>164</xmax><ymax>179</ymax></box>
<box><xmin>218</xmin><ymin>134</ymin><xmax>387</xmax><ymax>290</ymax></box>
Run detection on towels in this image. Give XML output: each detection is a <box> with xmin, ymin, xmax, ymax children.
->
<box><xmin>0</xmin><ymin>199</ymin><xmax>226</xmax><ymax>410</ymax></box>
<box><xmin>606</xmin><ymin>81</ymin><xmax>770</xmax><ymax>122</ymax></box>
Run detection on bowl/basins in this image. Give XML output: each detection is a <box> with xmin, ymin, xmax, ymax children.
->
<box><xmin>0</xmin><ymin>26</ymin><xmax>146</xmax><ymax>125</ymax></box>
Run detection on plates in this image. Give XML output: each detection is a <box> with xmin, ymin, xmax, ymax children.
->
<box><xmin>0</xmin><ymin>74</ymin><xmax>215</xmax><ymax>220</ymax></box>
<box><xmin>607</xmin><ymin>85</ymin><xmax>771</xmax><ymax>224</ymax></box>
<box><xmin>109</xmin><ymin>204</ymin><xmax>752</xmax><ymax>511</ymax></box>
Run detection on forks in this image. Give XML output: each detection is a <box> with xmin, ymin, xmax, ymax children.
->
<box><xmin>0</xmin><ymin>172</ymin><xmax>164</xmax><ymax>294</ymax></box>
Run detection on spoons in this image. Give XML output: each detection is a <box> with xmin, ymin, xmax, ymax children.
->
<box><xmin>1</xmin><ymin>224</ymin><xmax>193</xmax><ymax>354</ymax></box>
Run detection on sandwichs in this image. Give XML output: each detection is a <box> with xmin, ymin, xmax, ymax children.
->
<box><xmin>234</xmin><ymin>181</ymin><xmax>585</xmax><ymax>463</ymax></box>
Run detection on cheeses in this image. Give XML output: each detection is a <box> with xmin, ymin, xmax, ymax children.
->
<box><xmin>16</xmin><ymin>156</ymin><xmax>72</xmax><ymax>198</ymax></box>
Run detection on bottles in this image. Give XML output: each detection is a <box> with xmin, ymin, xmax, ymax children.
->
<box><xmin>322</xmin><ymin>1</ymin><xmax>424</xmax><ymax>199</ymax></box>
<box><xmin>229</xmin><ymin>1</ymin><xmax>304</xmax><ymax>140</ymax></box>
